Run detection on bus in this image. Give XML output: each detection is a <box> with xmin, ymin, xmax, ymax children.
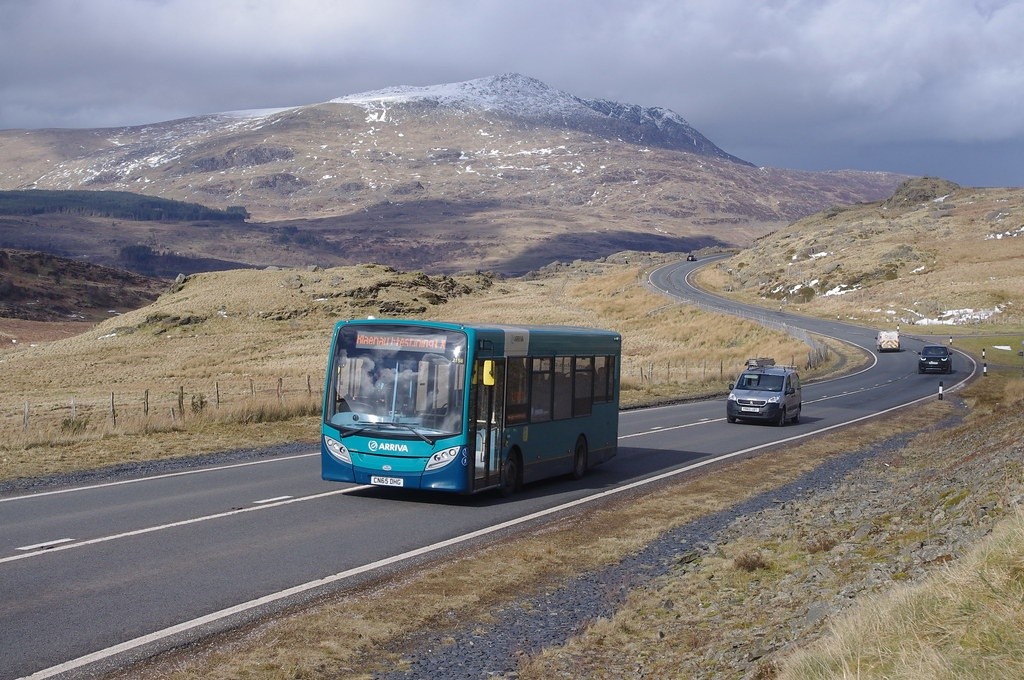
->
<box><xmin>321</xmin><ymin>316</ymin><xmax>622</xmax><ymax>500</ymax></box>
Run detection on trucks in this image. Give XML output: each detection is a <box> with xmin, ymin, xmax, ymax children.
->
<box><xmin>875</xmin><ymin>331</ymin><xmax>900</xmax><ymax>353</ymax></box>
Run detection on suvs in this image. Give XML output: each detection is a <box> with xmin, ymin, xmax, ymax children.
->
<box><xmin>726</xmin><ymin>365</ymin><xmax>801</xmax><ymax>427</ymax></box>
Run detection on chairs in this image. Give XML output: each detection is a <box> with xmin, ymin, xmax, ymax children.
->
<box><xmin>530</xmin><ymin>370</ymin><xmax>595</xmax><ymax>406</ymax></box>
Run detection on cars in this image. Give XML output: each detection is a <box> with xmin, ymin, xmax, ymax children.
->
<box><xmin>917</xmin><ymin>344</ymin><xmax>953</xmax><ymax>375</ymax></box>
